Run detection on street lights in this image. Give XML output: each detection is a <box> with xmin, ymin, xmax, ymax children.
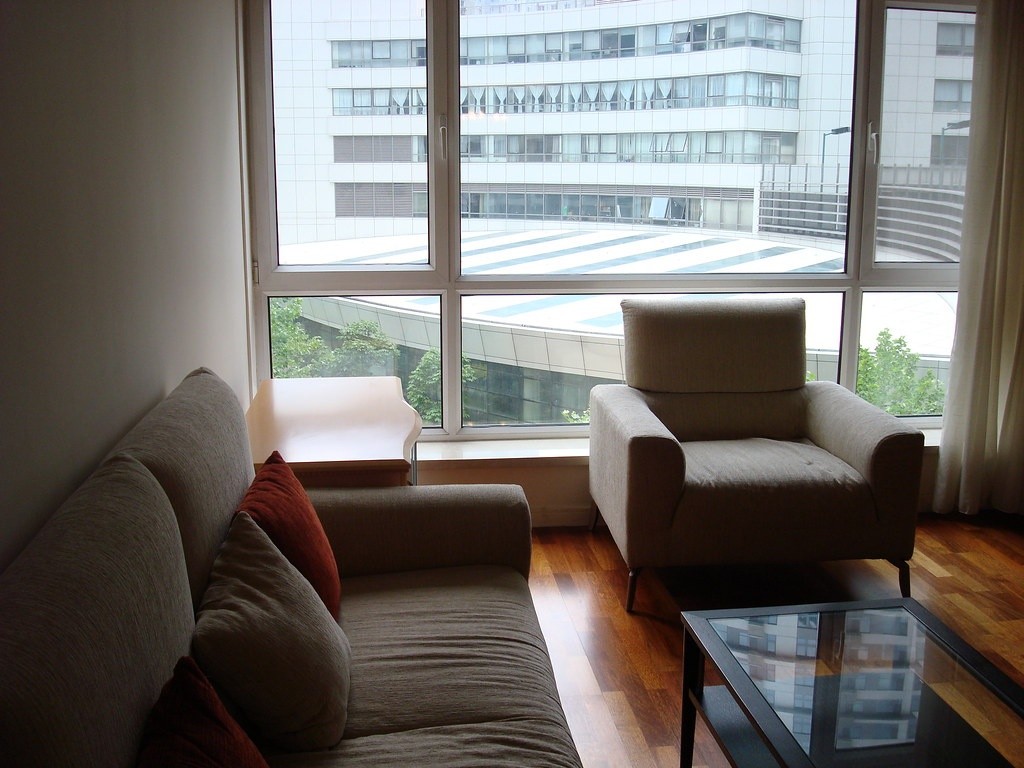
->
<box><xmin>935</xmin><ymin>117</ymin><xmax>970</xmax><ymax>187</ymax></box>
<box><xmin>817</xmin><ymin>125</ymin><xmax>852</xmax><ymax>235</ymax></box>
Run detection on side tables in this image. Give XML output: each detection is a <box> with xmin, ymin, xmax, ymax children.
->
<box><xmin>245</xmin><ymin>376</ymin><xmax>423</xmax><ymax>485</ymax></box>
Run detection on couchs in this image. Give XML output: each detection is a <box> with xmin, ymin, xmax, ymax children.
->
<box><xmin>588</xmin><ymin>300</ymin><xmax>924</xmax><ymax>612</ymax></box>
<box><xmin>0</xmin><ymin>367</ymin><xmax>584</xmax><ymax>768</ymax></box>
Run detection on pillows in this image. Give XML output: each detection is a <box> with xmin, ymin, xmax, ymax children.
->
<box><xmin>228</xmin><ymin>451</ymin><xmax>341</xmax><ymax>623</ymax></box>
<box><xmin>135</xmin><ymin>656</ymin><xmax>270</xmax><ymax>768</ymax></box>
<box><xmin>186</xmin><ymin>510</ymin><xmax>352</xmax><ymax>751</ymax></box>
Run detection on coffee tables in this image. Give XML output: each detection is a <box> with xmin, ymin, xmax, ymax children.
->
<box><xmin>680</xmin><ymin>598</ymin><xmax>1024</xmax><ymax>768</ymax></box>
